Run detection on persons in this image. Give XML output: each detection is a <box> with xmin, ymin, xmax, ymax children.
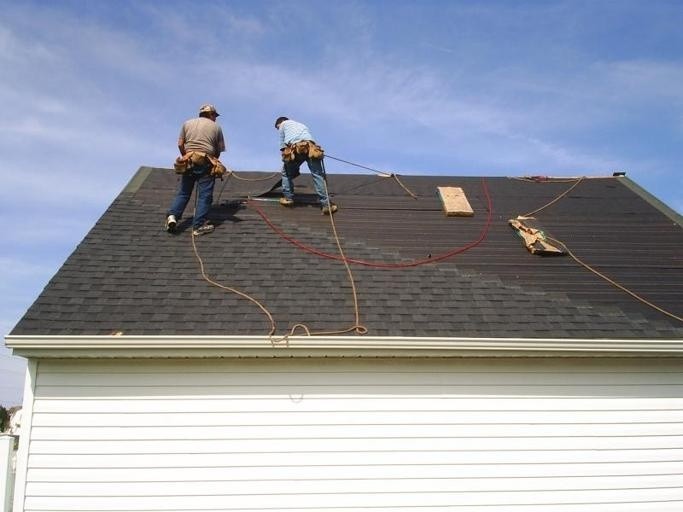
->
<box><xmin>164</xmin><ymin>104</ymin><xmax>227</xmax><ymax>237</ymax></box>
<box><xmin>275</xmin><ymin>116</ymin><xmax>337</xmax><ymax>216</ymax></box>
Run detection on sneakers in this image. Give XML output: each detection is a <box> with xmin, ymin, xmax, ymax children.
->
<box><xmin>193</xmin><ymin>224</ymin><xmax>215</xmax><ymax>236</ymax></box>
<box><xmin>165</xmin><ymin>215</ymin><xmax>177</xmax><ymax>232</ymax></box>
<box><xmin>280</xmin><ymin>197</ymin><xmax>293</xmax><ymax>206</ymax></box>
<box><xmin>322</xmin><ymin>205</ymin><xmax>337</xmax><ymax>215</ymax></box>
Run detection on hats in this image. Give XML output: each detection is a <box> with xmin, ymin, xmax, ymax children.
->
<box><xmin>199</xmin><ymin>103</ymin><xmax>220</xmax><ymax>116</ymax></box>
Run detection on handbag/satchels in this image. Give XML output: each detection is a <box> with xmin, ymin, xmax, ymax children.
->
<box><xmin>174</xmin><ymin>152</ymin><xmax>226</xmax><ymax>178</ymax></box>
<box><xmin>281</xmin><ymin>142</ymin><xmax>324</xmax><ymax>162</ymax></box>
